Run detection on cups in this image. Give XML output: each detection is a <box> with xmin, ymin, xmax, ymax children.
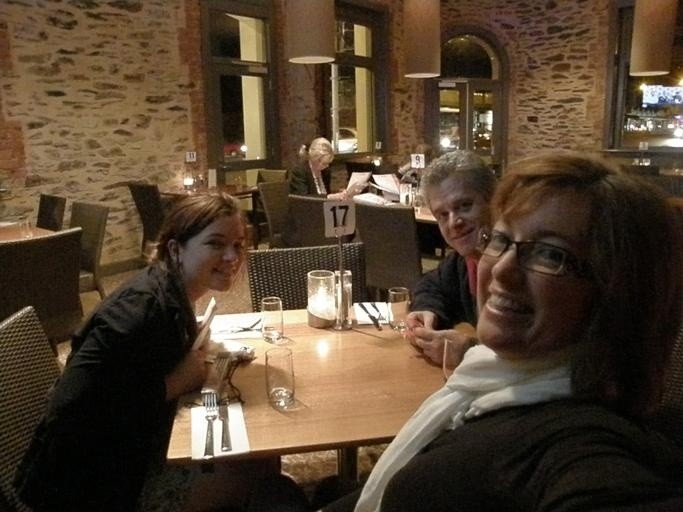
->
<box><xmin>638</xmin><ymin>141</ymin><xmax>649</xmax><ymax>152</ymax></box>
<box><xmin>398</xmin><ymin>183</ymin><xmax>413</xmax><ymax>208</ymax></box>
<box><xmin>639</xmin><ymin>158</ymin><xmax>650</xmax><ymax>166</ymax></box>
<box><xmin>19</xmin><ymin>215</ymin><xmax>34</xmax><ymax>240</ymax></box>
<box><xmin>265</xmin><ymin>346</ymin><xmax>296</xmax><ymax>411</ymax></box>
<box><xmin>306</xmin><ymin>270</ymin><xmax>336</xmax><ymax>328</ymax></box>
<box><xmin>386</xmin><ymin>286</ymin><xmax>411</xmax><ymax>334</ymax></box>
<box><xmin>260</xmin><ymin>296</ymin><xmax>285</xmax><ymax>343</ymax></box>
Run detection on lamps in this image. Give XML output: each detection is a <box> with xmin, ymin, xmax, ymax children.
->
<box><xmin>628</xmin><ymin>0</ymin><xmax>676</xmax><ymax>76</ymax></box>
<box><xmin>286</xmin><ymin>0</ymin><xmax>336</xmax><ymax>65</ymax></box>
<box><xmin>403</xmin><ymin>0</ymin><xmax>440</xmax><ymax>79</ymax></box>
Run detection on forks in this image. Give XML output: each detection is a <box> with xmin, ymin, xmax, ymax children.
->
<box><xmin>202</xmin><ymin>393</ymin><xmax>219</xmax><ymax>457</ymax></box>
<box><xmin>240</xmin><ymin>318</ymin><xmax>261</xmax><ymax>331</ymax></box>
<box><xmin>370</xmin><ymin>301</ymin><xmax>385</xmax><ymax>320</ymax></box>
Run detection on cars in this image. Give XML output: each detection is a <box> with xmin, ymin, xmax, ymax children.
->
<box><xmin>436</xmin><ymin>105</ymin><xmax>491</xmax><ymax>152</ymax></box>
<box><xmin>224</xmin><ymin>138</ymin><xmax>248</xmax><ymax>160</ymax></box>
<box><xmin>672</xmin><ymin>124</ymin><xmax>683</xmax><ymax>138</ymax></box>
<box><xmin>332</xmin><ymin>127</ymin><xmax>359</xmax><ymax>156</ymax></box>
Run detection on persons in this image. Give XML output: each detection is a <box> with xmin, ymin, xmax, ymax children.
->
<box><xmin>316</xmin><ymin>149</ymin><xmax>682</xmax><ymax>511</ymax></box>
<box><xmin>399</xmin><ymin>143</ymin><xmax>500</xmax><ymax>366</ymax></box>
<box><xmin>12</xmin><ymin>189</ymin><xmax>251</xmax><ymax>511</ymax></box>
<box><xmin>285</xmin><ymin>137</ymin><xmax>351</xmax><ymax>202</ymax></box>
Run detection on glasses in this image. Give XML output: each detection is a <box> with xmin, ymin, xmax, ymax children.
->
<box><xmin>476</xmin><ymin>227</ymin><xmax>599</xmax><ymax>279</ymax></box>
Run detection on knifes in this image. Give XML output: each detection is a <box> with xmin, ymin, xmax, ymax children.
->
<box><xmin>218</xmin><ymin>399</ymin><xmax>232</xmax><ymax>452</ymax></box>
<box><xmin>359</xmin><ymin>302</ymin><xmax>380</xmax><ymax>328</ymax></box>
